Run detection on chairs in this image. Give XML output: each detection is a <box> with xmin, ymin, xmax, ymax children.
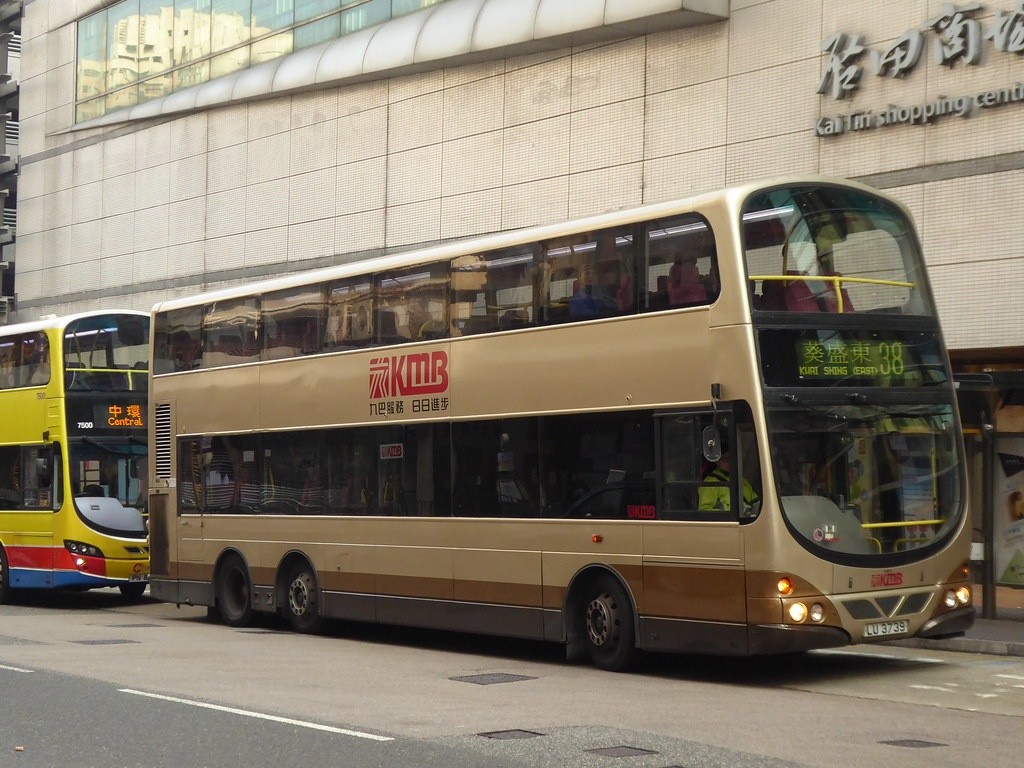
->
<box><xmin>336</xmin><ymin>316</ymin><xmax>372</xmax><ymax>346</ymax></box>
<box><xmin>111</xmin><ymin>364</ymin><xmax>131</xmax><ymax>388</ymax></box>
<box><xmin>418</xmin><ymin>319</ymin><xmax>450</xmax><ymax>339</ymax></box>
<box><xmin>762</xmin><ymin>280</ymin><xmax>784</xmax><ymax>311</ymax></box>
<box><xmin>537</xmin><ymin>302</ymin><xmax>570</xmax><ymax>323</ymax></box>
<box><xmin>616</xmin><ymin>271</ymin><xmax>634</xmax><ymax>312</ymax></box>
<box><xmin>212</xmin><ymin>335</ymin><xmax>243</xmax><ymax>356</ymax></box>
<box><xmin>502</xmin><ymin>310</ymin><xmax>529</xmax><ymax>326</ymax></box>
<box><xmin>822</xmin><ymin>272</ymin><xmax>855</xmax><ymax>313</ymax></box>
<box><xmin>780</xmin><ymin>270</ymin><xmax>821</xmax><ymax>311</ymax></box>
<box><xmin>466</xmin><ymin>315</ymin><xmax>499</xmax><ymax>334</ymax></box>
<box><xmin>302</xmin><ymin>320</ymin><xmax>325</xmax><ymax>353</ymax></box>
<box><xmin>667</xmin><ymin>264</ymin><xmax>706</xmax><ymax>305</ymax></box>
<box><xmin>652</xmin><ymin>276</ymin><xmax>669</xmax><ymax>307</ymax></box>
<box><xmin>408</xmin><ymin>311</ymin><xmax>433</xmax><ymax>339</ymax></box>
<box><xmin>271</xmin><ymin>325</ymin><xmax>302</xmax><ymax>348</ymax></box>
<box><xmin>246</xmin><ymin>331</ymin><xmax>272</xmax><ymax>356</ymax></box>
<box><xmin>371</xmin><ymin>310</ymin><xmax>410</xmax><ymax>344</ymax></box>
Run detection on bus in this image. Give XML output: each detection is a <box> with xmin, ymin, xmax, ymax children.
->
<box><xmin>147</xmin><ymin>174</ymin><xmax>1001</xmax><ymax>671</ymax></box>
<box><xmin>0</xmin><ymin>312</ymin><xmax>152</xmax><ymax>598</ymax></box>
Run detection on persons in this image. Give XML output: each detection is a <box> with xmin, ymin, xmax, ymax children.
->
<box><xmin>698</xmin><ymin>441</ymin><xmax>760</xmax><ymax>517</ymax></box>
<box><xmin>570</xmin><ymin>263</ymin><xmax>615</xmax><ymax>313</ymax></box>
